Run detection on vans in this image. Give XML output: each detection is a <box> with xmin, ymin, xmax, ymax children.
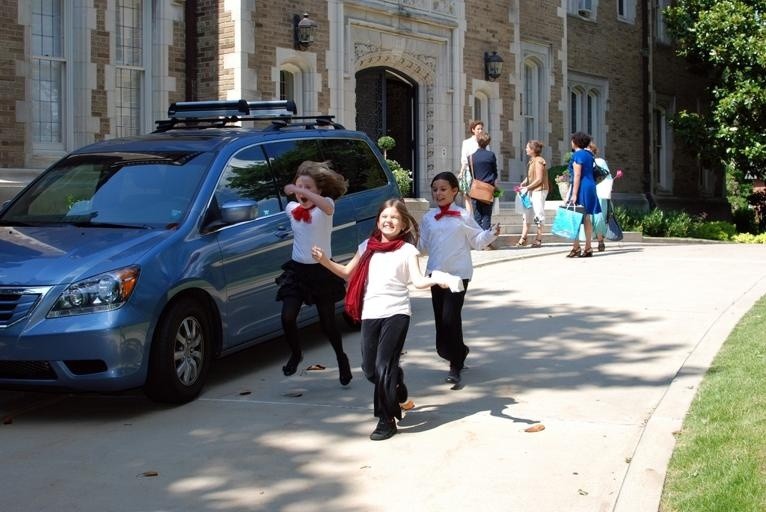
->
<box><xmin>1</xmin><ymin>99</ymin><xmax>408</xmax><ymax>404</ymax></box>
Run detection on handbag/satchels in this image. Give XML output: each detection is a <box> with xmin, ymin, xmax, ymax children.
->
<box><xmin>605</xmin><ymin>213</ymin><xmax>623</xmax><ymax>241</ymax></box>
<box><xmin>593</xmin><ymin>166</ymin><xmax>608</xmax><ymax>184</ymax></box>
<box><xmin>552</xmin><ymin>204</ymin><xmax>584</xmax><ymax>240</ymax></box>
<box><xmin>468</xmin><ymin>180</ymin><xmax>494</xmax><ymax>204</ymax></box>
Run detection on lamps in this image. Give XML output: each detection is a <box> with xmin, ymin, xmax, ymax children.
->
<box><xmin>293</xmin><ymin>12</ymin><xmax>316</xmax><ymax>53</ymax></box>
<box><xmin>484</xmin><ymin>49</ymin><xmax>504</xmax><ymax>83</ymax></box>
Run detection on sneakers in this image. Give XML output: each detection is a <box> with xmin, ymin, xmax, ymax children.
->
<box><xmin>598</xmin><ymin>240</ymin><xmax>605</xmax><ymax>252</ymax></box>
<box><xmin>337</xmin><ymin>353</ymin><xmax>352</xmax><ymax>385</ymax></box>
<box><xmin>370</xmin><ymin>419</ymin><xmax>397</xmax><ymax>440</ymax></box>
<box><xmin>395</xmin><ymin>367</ymin><xmax>407</xmax><ymax>403</ymax></box>
<box><xmin>444</xmin><ymin>346</ymin><xmax>469</xmax><ymax>383</ymax></box>
<box><xmin>282</xmin><ymin>356</ymin><xmax>301</xmax><ymax>376</ymax></box>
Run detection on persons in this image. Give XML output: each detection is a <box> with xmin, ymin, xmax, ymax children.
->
<box><xmin>584</xmin><ymin>142</ymin><xmax>614</xmax><ymax>252</ymax></box>
<box><xmin>513</xmin><ymin>139</ymin><xmax>551</xmax><ymax>248</ymax></box>
<box><xmin>274</xmin><ymin>160</ymin><xmax>352</xmax><ymax>386</ymax></box>
<box><xmin>416</xmin><ymin>171</ymin><xmax>502</xmax><ymax>384</ymax></box>
<box><xmin>460</xmin><ymin>131</ymin><xmax>498</xmax><ymax>251</ymax></box>
<box><xmin>311</xmin><ymin>196</ymin><xmax>465</xmax><ymax>440</ymax></box>
<box><xmin>565</xmin><ymin>132</ymin><xmax>603</xmax><ymax>258</ymax></box>
<box><xmin>456</xmin><ymin>120</ymin><xmax>493</xmax><ymax>214</ymax></box>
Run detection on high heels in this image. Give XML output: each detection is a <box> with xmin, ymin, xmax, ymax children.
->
<box><xmin>516</xmin><ymin>236</ymin><xmax>541</xmax><ymax>248</ymax></box>
<box><xmin>567</xmin><ymin>247</ymin><xmax>593</xmax><ymax>257</ymax></box>
